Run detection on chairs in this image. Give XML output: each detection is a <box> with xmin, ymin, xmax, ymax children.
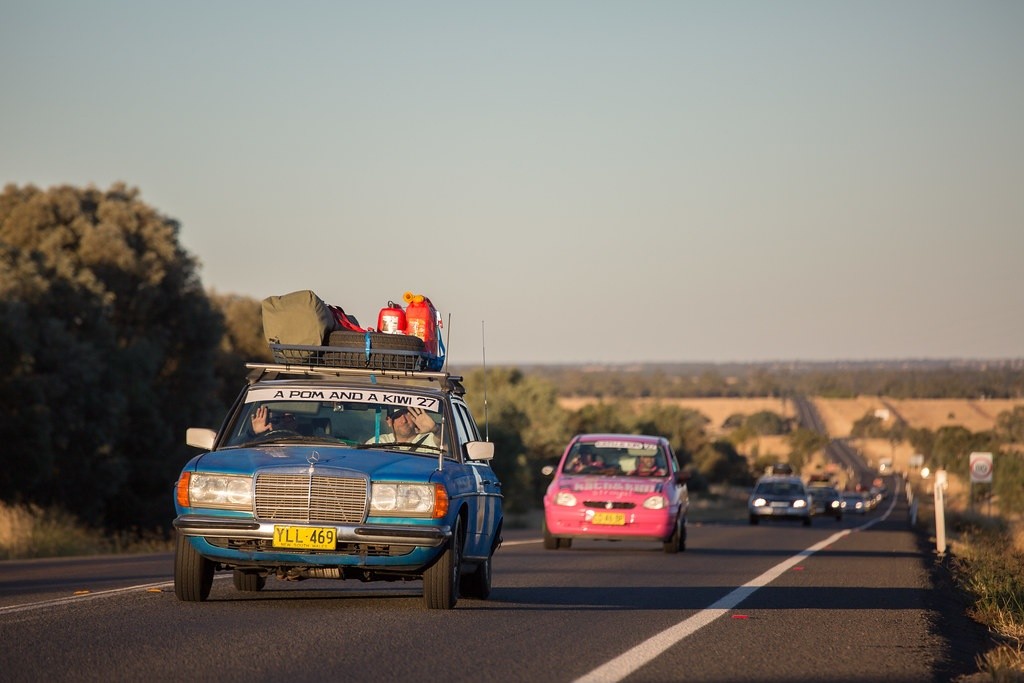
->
<box><xmin>593</xmin><ymin>455</ymin><xmax>607</xmax><ymax>468</ymax></box>
<box><xmin>296</xmin><ymin>417</ymin><xmax>333</xmax><ymax>438</ymax></box>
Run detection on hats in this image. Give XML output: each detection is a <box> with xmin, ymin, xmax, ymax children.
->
<box><xmin>387</xmin><ymin>405</ymin><xmax>409</xmax><ymax>419</ymax></box>
<box><xmin>578</xmin><ymin>445</ymin><xmax>595</xmax><ymax>454</ymax></box>
<box><xmin>280</xmin><ymin>409</ymin><xmax>296</xmax><ymax>421</ymax></box>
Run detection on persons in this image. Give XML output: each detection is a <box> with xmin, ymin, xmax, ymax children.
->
<box><xmin>565</xmin><ymin>448</ymin><xmax>607</xmax><ymax>475</ymax></box>
<box><xmin>230</xmin><ymin>404</ymin><xmax>304</xmax><ymax>446</ymax></box>
<box><xmin>365</xmin><ymin>405</ymin><xmax>449</xmax><ymax>456</ymax></box>
<box><xmin>626</xmin><ymin>456</ymin><xmax>665</xmax><ymax>477</ymax></box>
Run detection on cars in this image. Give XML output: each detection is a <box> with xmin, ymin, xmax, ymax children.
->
<box><xmin>838</xmin><ymin>483</ymin><xmax>889</xmax><ymax>516</ymax></box>
<box><xmin>745</xmin><ymin>472</ymin><xmax>817</xmax><ymax>527</ymax></box>
<box><xmin>541</xmin><ymin>433</ymin><xmax>692</xmax><ymax>555</ymax></box>
<box><xmin>172</xmin><ymin>340</ymin><xmax>505</xmax><ymax>611</ymax></box>
<box><xmin>806</xmin><ymin>485</ymin><xmax>846</xmax><ymax>521</ymax></box>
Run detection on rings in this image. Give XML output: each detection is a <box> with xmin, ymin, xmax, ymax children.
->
<box><xmin>418</xmin><ymin>413</ymin><xmax>421</xmax><ymax>415</ymax></box>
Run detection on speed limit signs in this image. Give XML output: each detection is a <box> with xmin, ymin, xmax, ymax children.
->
<box><xmin>971</xmin><ymin>456</ymin><xmax>994</xmax><ymax>480</ymax></box>
